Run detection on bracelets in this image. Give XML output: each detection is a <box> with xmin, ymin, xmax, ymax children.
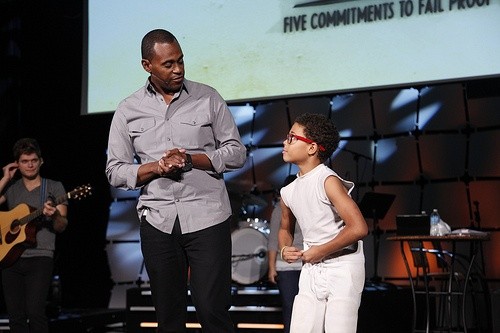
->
<box><xmin>280</xmin><ymin>245</ymin><xmax>288</xmax><ymax>261</ymax></box>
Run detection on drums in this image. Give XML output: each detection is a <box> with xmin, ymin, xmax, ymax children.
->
<box><xmin>230</xmin><ymin>221</ymin><xmax>271</xmax><ymax>287</ymax></box>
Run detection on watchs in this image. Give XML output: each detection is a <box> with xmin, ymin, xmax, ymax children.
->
<box><xmin>183</xmin><ymin>153</ymin><xmax>193</xmax><ymax>171</ymax></box>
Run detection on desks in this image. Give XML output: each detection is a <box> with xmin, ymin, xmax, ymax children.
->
<box><xmin>384</xmin><ymin>232</ymin><xmax>486</xmax><ymax>333</ymax></box>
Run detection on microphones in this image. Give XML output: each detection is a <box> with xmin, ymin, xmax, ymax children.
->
<box><xmin>259</xmin><ymin>252</ymin><xmax>265</xmax><ymax>258</ymax></box>
<box><xmin>152</xmin><ymin>74</ymin><xmax>168</xmax><ymax>84</ymax></box>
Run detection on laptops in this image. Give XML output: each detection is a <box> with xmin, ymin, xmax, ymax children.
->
<box><xmin>396</xmin><ymin>214</ymin><xmax>431</xmax><ymax>236</ymax></box>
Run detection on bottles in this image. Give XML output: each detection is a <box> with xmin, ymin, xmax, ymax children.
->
<box><xmin>430</xmin><ymin>209</ymin><xmax>440</xmax><ymax>235</ymax></box>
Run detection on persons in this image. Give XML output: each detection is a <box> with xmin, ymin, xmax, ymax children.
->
<box><xmin>277</xmin><ymin>112</ymin><xmax>369</xmax><ymax>333</ymax></box>
<box><xmin>0</xmin><ymin>137</ymin><xmax>68</xmax><ymax>333</ymax></box>
<box><xmin>105</xmin><ymin>29</ymin><xmax>247</xmax><ymax>333</ymax></box>
<box><xmin>431</xmin><ymin>224</ymin><xmax>482</xmax><ymax>333</ymax></box>
<box><xmin>268</xmin><ymin>174</ymin><xmax>304</xmax><ymax>333</ymax></box>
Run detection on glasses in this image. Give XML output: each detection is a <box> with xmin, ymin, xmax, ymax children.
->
<box><xmin>286</xmin><ymin>132</ymin><xmax>326</xmax><ymax>151</ymax></box>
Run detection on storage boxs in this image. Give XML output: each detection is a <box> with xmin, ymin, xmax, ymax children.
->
<box><xmin>396</xmin><ymin>215</ymin><xmax>431</xmax><ymax>236</ymax></box>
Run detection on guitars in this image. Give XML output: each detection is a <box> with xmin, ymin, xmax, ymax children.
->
<box><xmin>0</xmin><ymin>184</ymin><xmax>92</xmax><ymax>269</ymax></box>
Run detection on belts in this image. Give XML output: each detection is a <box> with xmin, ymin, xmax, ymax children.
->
<box><xmin>324</xmin><ymin>243</ymin><xmax>358</xmax><ymax>264</ymax></box>
